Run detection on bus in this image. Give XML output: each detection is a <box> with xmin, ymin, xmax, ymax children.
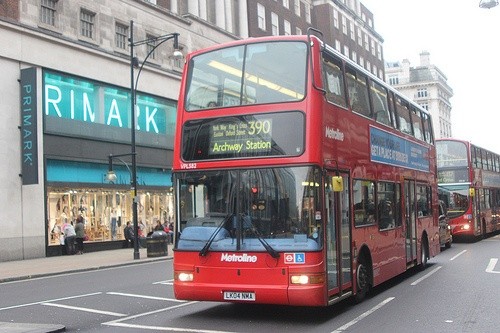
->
<box><xmin>171</xmin><ymin>28</ymin><xmax>440</xmax><ymax>308</ymax></box>
<box><xmin>436</xmin><ymin>138</ymin><xmax>500</xmax><ymax>242</ymax></box>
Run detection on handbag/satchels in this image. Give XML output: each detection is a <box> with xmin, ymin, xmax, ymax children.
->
<box><xmin>83</xmin><ymin>235</ymin><xmax>89</xmax><ymax>240</ymax></box>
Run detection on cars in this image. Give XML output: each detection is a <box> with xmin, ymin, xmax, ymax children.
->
<box><xmin>438</xmin><ymin>200</ymin><xmax>453</xmax><ymax>248</ymax></box>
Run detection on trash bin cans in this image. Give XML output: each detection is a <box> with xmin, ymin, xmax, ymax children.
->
<box><xmin>147</xmin><ymin>231</ymin><xmax>168</xmax><ymax>258</ymax></box>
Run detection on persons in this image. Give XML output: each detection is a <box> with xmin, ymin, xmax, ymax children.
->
<box><xmin>110</xmin><ymin>209</ymin><xmax>117</xmax><ymax>238</ymax></box>
<box><xmin>124</xmin><ymin>219</ymin><xmax>175</xmax><ymax>249</ymax></box>
<box><xmin>62</xmin><ymin>206</ymin><xmax>85</xmax><ymax>255</ymax></box>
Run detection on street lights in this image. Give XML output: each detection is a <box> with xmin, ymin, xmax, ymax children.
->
<box><xmin>107</xmin><ymin>30</ymin><xmax>185</xmax><ymax>260</ymax></box>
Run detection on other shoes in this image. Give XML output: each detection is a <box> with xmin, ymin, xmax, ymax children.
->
<box><xmin>76</xmin><ymin>250</ymin><xmax>83</xmax><ymax>255</ymax></box>
